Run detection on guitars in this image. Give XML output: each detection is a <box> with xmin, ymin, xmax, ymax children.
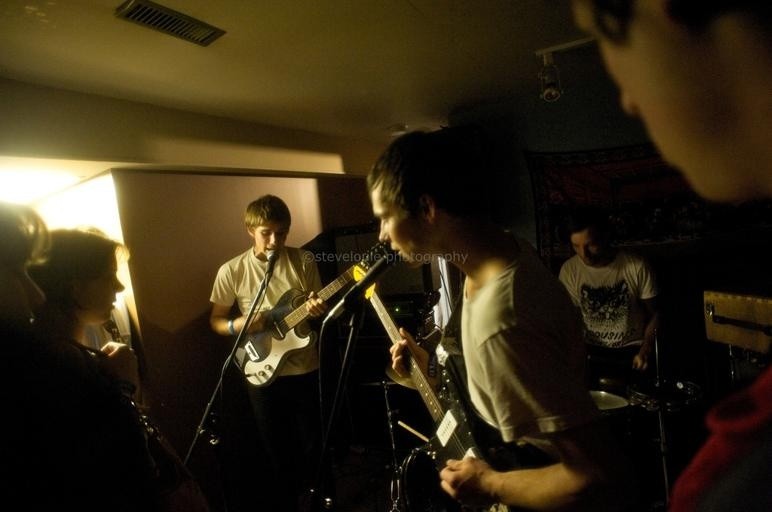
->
<box><xmin>364</xmin><ymin>282</ymin><xmax>545</xmax><ymax>512</ymax></box>
<box><xmin>232</xmin><ymin>242</ymin><xmax>395</xmax><ymax>387</ymax></box>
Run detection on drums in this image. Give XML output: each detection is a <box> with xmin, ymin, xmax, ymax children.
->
<box><xmin>623</xmin><ymin>373</ymin><xmax>696</xmax><ymax>441</ymax></box>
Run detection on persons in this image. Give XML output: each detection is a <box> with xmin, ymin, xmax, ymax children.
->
<box><xmin>1</xmin><ymin>128</ymin><xmax>661</xmax><ymax>512</ymax></box>
<box><xmin>568</xmin><ymin>1</ymin><xmax>767</xmax><ymax>512</ymax></box>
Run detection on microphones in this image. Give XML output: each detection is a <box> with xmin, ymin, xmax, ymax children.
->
<box><xmin>266</xmin><ymin>251</ymin><xmax>279</xmax><ymax>280</ymax></box>
<box><xmin>326</xmin><ymin>252</ymin><xmax>388</xmax><ymax>319</ymax></box>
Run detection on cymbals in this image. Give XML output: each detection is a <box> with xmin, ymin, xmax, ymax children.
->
<box><xmin>591</xmin><ymin>391</ymin><xmax>628</xmax><ymax>411</ymax></box>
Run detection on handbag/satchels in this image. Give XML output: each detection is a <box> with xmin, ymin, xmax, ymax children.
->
<box><xmin>132</xmin><ymin>403</ymin><xmax>187</xmax><ymax>498</ymax></box>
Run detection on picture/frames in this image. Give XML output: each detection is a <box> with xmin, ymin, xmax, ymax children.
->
<box><xmin>327</xmin><ymin>222</ymin><xmax>434</xmax><ymax>305</ymax></box>
